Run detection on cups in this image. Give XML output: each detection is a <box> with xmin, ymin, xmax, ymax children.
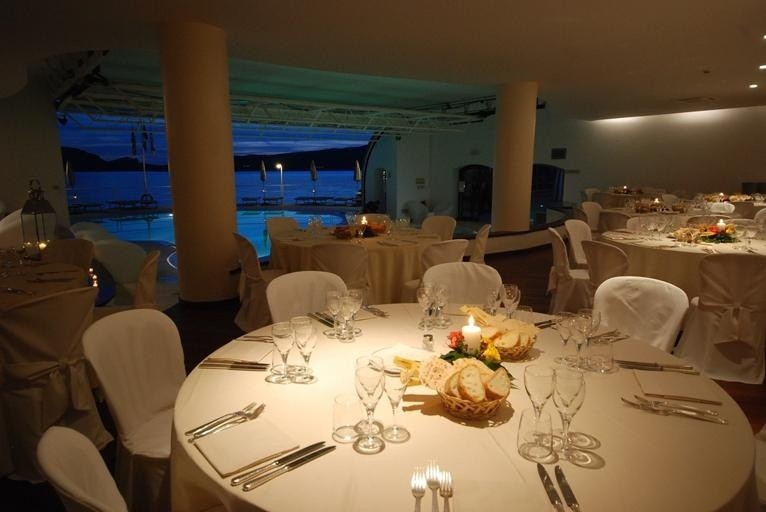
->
<box><xmin>270</xmin><ymin>344</ymin><xmax>293</xmax><ymax>376</ymax></box>
<box><xmin>517</xmin><ymin>408</ymin><xmax>553</xmax><ymax>461</ymax></box>
<box><xmin>588</xmin><ymin>336</ymin><xmax>614</xmax><ymax>372</ymax></box>
<box><xmin>516</xmin><ymin>305</ymin><xmax>532</xmax><ymax>324</ymax></box>
<box><xmin>332</xmin><ymin>394</ymin><xmax>361</xmax><ymax>444</ymax></box>
<box><xmin>335</xmin><ymin>316</ymin><xmax>355</xmax><ymax>342</ymax></box>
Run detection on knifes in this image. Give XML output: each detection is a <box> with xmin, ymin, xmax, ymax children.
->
<box><xmin>612</xmin><ymin>357</ymin><xmax>702</xmax><ymax>376</ymax></box>
<box><xmin>537</xmin><ymin>319</ymin><xmax>563</xmax><ymax>329</ymax></box>
<box><xmin>307</xmin><ymin>313</ymin><xmax>335</xmax><ymax>328</ymax></box>
<box><xmin>535</xmin><ymin>463</ymin><xmax>582</xmax><ymax>512</ymax></box>
<box><xmin>316</xmin><ymin>311</ymin><xmax>340</xmax><ymax>325</ymax></box>
<box><xmin>231</xmin><ymin>440</ymin><xmax>337</xmax><ymax>492</ymax></box>
<box><xmin>199</xmin><ymin>359</ymin><xmax>268</xmax><ymax>372</ymax></box>
<box><xmin>534</xmin><ymin>318</ymin><xmax>566</xmax><ymax>326</ymax></box>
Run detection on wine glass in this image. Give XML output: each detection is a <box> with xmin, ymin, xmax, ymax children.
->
<box><xmin>354</xmin><ymin>355</ymin><xmax>384</xmax><ymax>436</ymax></box>
<box><xmin>338</xmin><ymin>296</ymin><xmax>353</xmax><ymax>340</ymax></box>
<box><xmin>303</xmin><ymin>210</ymin><xmax>412</xmax><ymax>247</ymax></box>
<box><xmin>353</xmin><ymin>367</ymin><xmax>385</xmax><ymax>455</ymax></box>
<box><xmin>433</xmin><ymin>287</ymin><xmax>449</xmax><ymax>329</ymax></box>
<box><xmin>555</xmin><ymin>312</ymin><xmax>575</xmax><ymax>363</ymax></box>
<box><xmin>499</xmin><ymin>284</ymin><xmax>518</xmax><ymax>316</ymax></box>
<box><xmin>380</xmin><ymin>368</ymin><xmax>410</xmax><ymax>443</ymax></box>
<box><xmin>504</xmin><ymin>290</ymin><xmax>520</xmax><ymax>319</ymax></box>
<box><xmin>272</xmin><ymin>323</ymin><xmax>295</xmax><ymax>384</ymax></box>
<box><xmin>633</xmin><ymin>205</ymin><xmax>764</xmax><ymax>254</ymax></box>
<box><xmin>523</xmin><ymin>364</ymin><xmax>557</xmax><ymax>445</ymax></box>
<box><xmin>291</xmin><ymin>326</ymin><xmax>317</xmax><ymax>383</ymax></box>
<box><xmin>327</xmin><ymin>288</ymin><xmax>342</xmax><ymax>339</ymax></box>
<box><xmin>488</xmin><ymin>286</ymin><xmax>503</xmax><ymax>316</ymax></box>
<box><xmin>289</xmin><ymin>316</ymin><xmax>312</xmax><ymax>375</ymax></box>
<box><xmin>577</xmin><ymin>308</ymin><xmax>601</xmax><ymax>366</ymax></box>
<box><xmin>553</xmin><ymin>367</ymin><xmax>583</xmax><ymax>452</ymax></box>
<box><xmin>416</xmin><ymin>287</ymin><xmax>434</xmax><ymax>336</ymax></box>
<box><xmin>551</xmin><ymin>374</ymin><xmax>587</xmax><ymax>461</ymax></box>
<box><xmin>0</xmin><ymin>240</ymin><xmax>53</xmax><ymax>280</ymax></box>
<box><xmin>568</xmin><ymin>315</ymin><xmax>591</xmax><ymax>371</ymax></box>
<box><xmin>345</xmin><ymin>289</ymin><xmax>364</xmax><ymax>335</ymax></box>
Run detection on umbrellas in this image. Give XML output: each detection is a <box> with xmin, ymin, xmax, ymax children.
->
<box><xmin>354</xmin><ymin>159</ymin><xmax>362</xmax><ymax>203</ymax></box>
<box><xmin>260</xmin><ymin>160</ymin><xmax>268</xmax><ymax>202</ymax></box>
<box><xmin>311</xmin><ymin>160</ymin><xmax>317</xmax><ymax>204</ymax></box>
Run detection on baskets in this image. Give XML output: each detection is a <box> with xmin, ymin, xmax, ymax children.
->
<box><xmin>437</xmin><ymin>387</ymin><xmax>509</xmax><ymax>420</ymax></box>
<box><xmin>481</xmin><ymin>339</ymin><xmax>536</xmax><ymax>361</ymax></box>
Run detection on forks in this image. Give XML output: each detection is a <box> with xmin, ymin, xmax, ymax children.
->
<box><xmin>409</xmin><ymin>459</ymin><xmax>453</xmax><ymax>512</ymax></box>
<box><xmin>621</xmin><ymin>394</ymin><xmax>730</xmax><ymax>424</ymax></box>
<box><xmin>182</xmin><ymin>401</ymin><xmax>265</xmax><ymax>445</ymax></box>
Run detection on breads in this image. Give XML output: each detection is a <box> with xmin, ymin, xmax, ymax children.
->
<box><xmin>445</xmin><ymin>364</ymin><xmax>511</xmax><ymax>404</ymax></box>
<box><xmin>491</xmin><ymin>332</ymin><xmax>531</xmax><ymax>348</ymax></box>
